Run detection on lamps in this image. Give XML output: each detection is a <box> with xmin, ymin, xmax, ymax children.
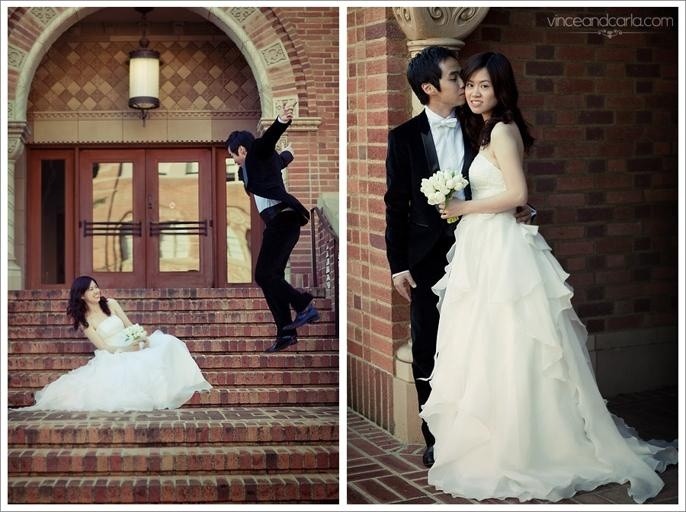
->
<box><xmin>125</xmin><ymin>7</ymin><xmax>163</xmax><ymax>114</ymax></box>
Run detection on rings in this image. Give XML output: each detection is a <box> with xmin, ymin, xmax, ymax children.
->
<box><xmin>529</xmin><ymin>214</ymin><xmax>532</xmax><ymax>218</ymax></box>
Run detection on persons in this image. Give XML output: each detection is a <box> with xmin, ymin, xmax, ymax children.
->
<box><xmin>226</xmin><ymin>102</ymin><xmax>320</xmax><ymax>354</ymax></box>
<box><xmin>30</xmin><ymin>277</ymin><xmax>212</xmax><ymax>413</ymax></box>
<box><xmin>382</xmin><ymin>47</ymin><xmax>538</xmax><ymax>468</ymax></box>
<box><xmin>417</xmin><ymin>50</ymin><xmax>676</xmax><ymax>502</ymax></box>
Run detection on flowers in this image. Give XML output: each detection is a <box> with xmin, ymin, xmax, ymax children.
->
<box><xmin>418</xmin><ymin>169</ymin><xmax>468</xmax><ymax>223</ymax></box>
<box><xmin>122</xmin><ymin>323</ymin><xmax>147</xmax><ymax>345</ymax></box>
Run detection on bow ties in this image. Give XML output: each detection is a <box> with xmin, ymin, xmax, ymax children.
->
<box><xmin>429</xmin><ymin>117</ymin><xmax>458</xmax><ymax>130</ymax></box>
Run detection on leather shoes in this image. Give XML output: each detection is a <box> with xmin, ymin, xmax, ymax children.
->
<box><xmin>422</xmin><ymin>446</ymin><xmax>435</xmax><ymax>469</ymax></box>
<box><xmin>265</xmin><ymin>335</ymin><xmax>298</xmax><ymax>354</ymax></box>
<box><xmin>283</xmin><ymin>305</ymin><xmax>320</xmax><ymax>331</ymax></box>
